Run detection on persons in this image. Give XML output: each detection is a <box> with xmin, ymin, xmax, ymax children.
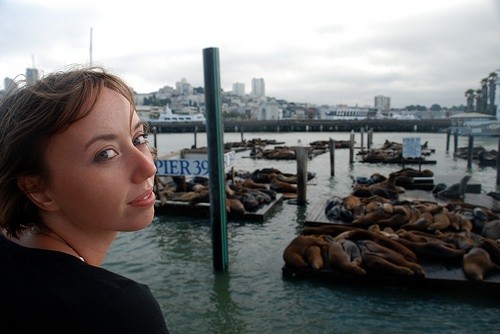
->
<box><xmin>0</xmin><ymin>70</ymin><xmax>169</xmax><ymax>334</ymax></box>
